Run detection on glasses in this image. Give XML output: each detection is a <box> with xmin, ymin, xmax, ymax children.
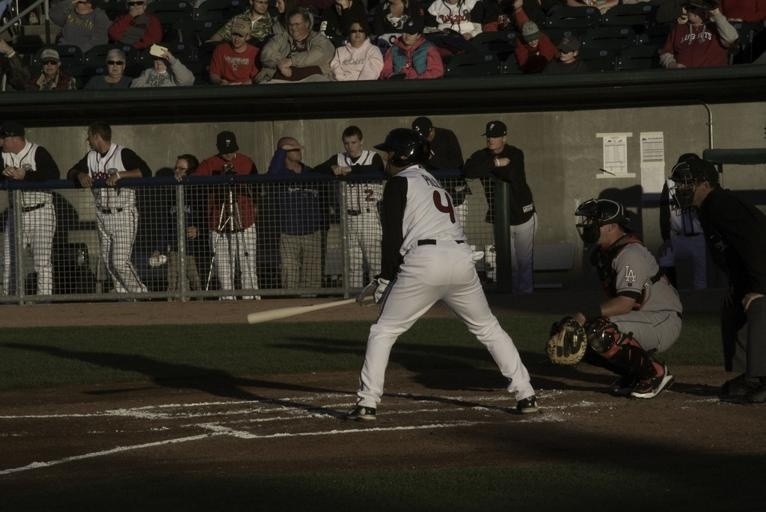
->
<box><xmin>350</xmin><ymin>29</ymin><xmax>365</xmax><ymax>32</ymax></box>
<box><xmin>108</xmin><ymin>60</ymin><xmax>123</xmax><ymax>65</ymax></box>
<box><xmin>41</xmin><ymin>59</ymin><xmax>59</xmax><ymax>65</ymax></box>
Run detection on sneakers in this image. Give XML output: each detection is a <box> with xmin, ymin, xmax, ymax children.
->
<box><xmin>718</xmin><ymin>372</ymin><xmax>766</xmax><ymax>405</ymax></box>
<box><xmin>347</xmin><ymin>407</ymin><xmax>376</xmax><ymax>421</ymax></box>
<box><xmin>630</xmin><ymin>364</ymin><xmax>673</xmax><ymax>399</ymax></box>
<box><xmin>518</xmin><ymin>395</ymin><xmax>538</xmax><ymax>414</ymax></box>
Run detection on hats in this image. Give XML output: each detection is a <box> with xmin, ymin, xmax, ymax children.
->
<box><xmin>40</xmin><ymin>49</ymin><xmax>59</xmax><ymax>58</ymax></box>
<box><xmin>411</xmin><ymin>117</ymin><xmax>433</xmax><ymax>137</ymax></box>
<box><xmin>522</xmin><ymin>21</ymin><xmax>541</xmax><ymax>42</ymax></box>
<box><xmin>230</xmin><ymin>15</ymin><xmax>251</xmax><ymax>36</ymax></box>
<box><xmin>481</xmin><ymin>120</ymin><xmax>507</xmax><ymax>138</ymax></box>
<box><xmin>217</xmin><ymin>131</ymin><xmax>239</xmax><ymax>154</ymax></box>
<box><xmin>556</xmin><ymin>35</ymin><xmax>579</xmax><ymax>51</ymax></box>
<box><xmin>400</xmin><ymin>20</ymin><xmax>418</xmax><ymax>35</ymax></box>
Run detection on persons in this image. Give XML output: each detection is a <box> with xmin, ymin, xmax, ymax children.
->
<box><xmin>266</xmin><ymin>134</ymin><xmax>326</xmax><ymax>299</ymax></box>
<box><xmin>195</xmin><ymin>129</ymin><xmax>265</xmax><ymax>304</ymax></box>
<box><xmin>411</xmin><ymin>114</ymin><xmax>468</xmax><ymax>232</ymax></box>
<box><xmin>68</xmin><ymin>123</ymin><xmax>155</xmax><ymax>303</ymax></box>
<box><xmin>314</xmin><ymin>125</ymin><xmax>384</xmax><ymax>300</ymax></box>
<box><xmin>2</xmin><ymin>118</ymin><xmax>61</xmax><ymax>305</ymax></box>
<box><xmin>463</xmin><ymin>117</ymin><xmax>538</xmax><ymax>299</ymax></box>
<box><xmin>1</xmin><ymin>1</ymin><xmax>765</xmax><ymax>94</ymax></box>
<box><xmin>549</xmin><ymin>199</ymin><xmax>685</xmax><ymax>402</ymax></box>
<box><xmin>155</xmin><ymin>150</ymin><xmax>205</xmax><ymax>301</ymax></box>
<box><xmin>655</xmin><ymin>153</ymin><xmax>714</xmax><ymax>293</ymax></box>
<box><xmin>669</xmin><ymin>157</ymin><xmax>766</xmax><ymax>405</ymax></box>
<box><xmin>348</xmin><ymin>127</ymin><xmax>542</xmax><ymax>422</ymax></box>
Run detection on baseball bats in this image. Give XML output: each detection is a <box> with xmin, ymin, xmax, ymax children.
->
<box><xmin>247</xmin><ymin>296</ymin><xmax>375</xmax><ymax>324</ymax></box>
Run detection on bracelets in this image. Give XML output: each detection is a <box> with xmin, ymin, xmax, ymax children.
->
<box><xmin>580</xmin><ymin>303</ymin><xmax>603</xmax><ymax>322</ymax></box>
<box><xmin>113</xmin><ymin>171</ymin><xmax>125</xmax><ymax>181</ymax></box>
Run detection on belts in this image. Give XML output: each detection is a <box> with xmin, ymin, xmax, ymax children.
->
<box><xmin>418</xmin><ymin>239</ymin><xmax>464</xmax><ymax>246</ymax></box>
<box><xmin>96</xmin><ymin>206</ymin><xmax>123</xmax><ymax>213</ymax></box>
<box><xmin>22</xmin><ymin>202</ymin><xmax>44</xmax><ymax>211</ymax></box>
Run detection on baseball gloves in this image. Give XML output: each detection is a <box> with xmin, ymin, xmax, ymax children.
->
<box><xmin>548</xmin><ymin>317</ymin><xmax>588</xmax><ymax>366</ymax></box>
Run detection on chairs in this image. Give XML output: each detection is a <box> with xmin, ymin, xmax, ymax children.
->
<box><xmin>14</xmin><ymin>2</ymin><xmax>752</xmax><ymax>92</ymax></box>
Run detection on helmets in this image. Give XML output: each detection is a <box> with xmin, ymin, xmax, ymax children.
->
<box><xmin>666</xmin><ymin>161</ymin><xmax>695</xmax><ymax>216</ymax></box>
<box><xmin>374</xmin><ymin>128</ymin><xmax>431</xmax><ymax>167</ymax></box>
<box><xmin>575</xmin><ymin>198</ymin><xmax>626</xmax><ymax>245</ymax></box>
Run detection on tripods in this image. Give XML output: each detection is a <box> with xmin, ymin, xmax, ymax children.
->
<box><xmin>202</xmin><ymin>190</ymin><xmax>259</xmax><ymax>300</ymax></box>
